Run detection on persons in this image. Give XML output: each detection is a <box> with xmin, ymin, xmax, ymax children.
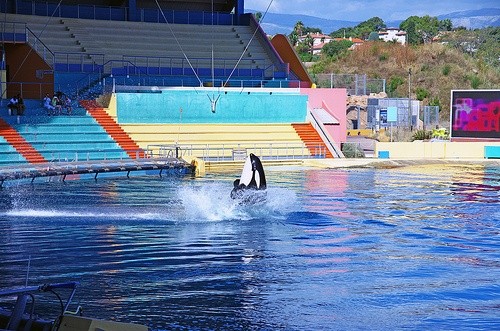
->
<box><xmin>8</xmin><ymin>94</ymin><xmax>25</xmax><ymax>115</ymax></box>
<box><xmin>230</xmin><ymin>179</ymin><xmax>246</xmax><ymax>199</ymax></box>
<box><xmin>43</xmin><ymin>94</ymin><xmax>72</xmax><ymax>116</ymax></box>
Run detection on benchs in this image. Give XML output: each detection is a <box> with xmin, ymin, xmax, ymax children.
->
<box><xmin>0</xmin><ymin>98</ymin><xmax>132</xmax><ymax>166</ymax></box>
<box><xmin>0</xmin><ymin>13</ymin><xmax>286</xmax><ymax>79</ymax></box>
